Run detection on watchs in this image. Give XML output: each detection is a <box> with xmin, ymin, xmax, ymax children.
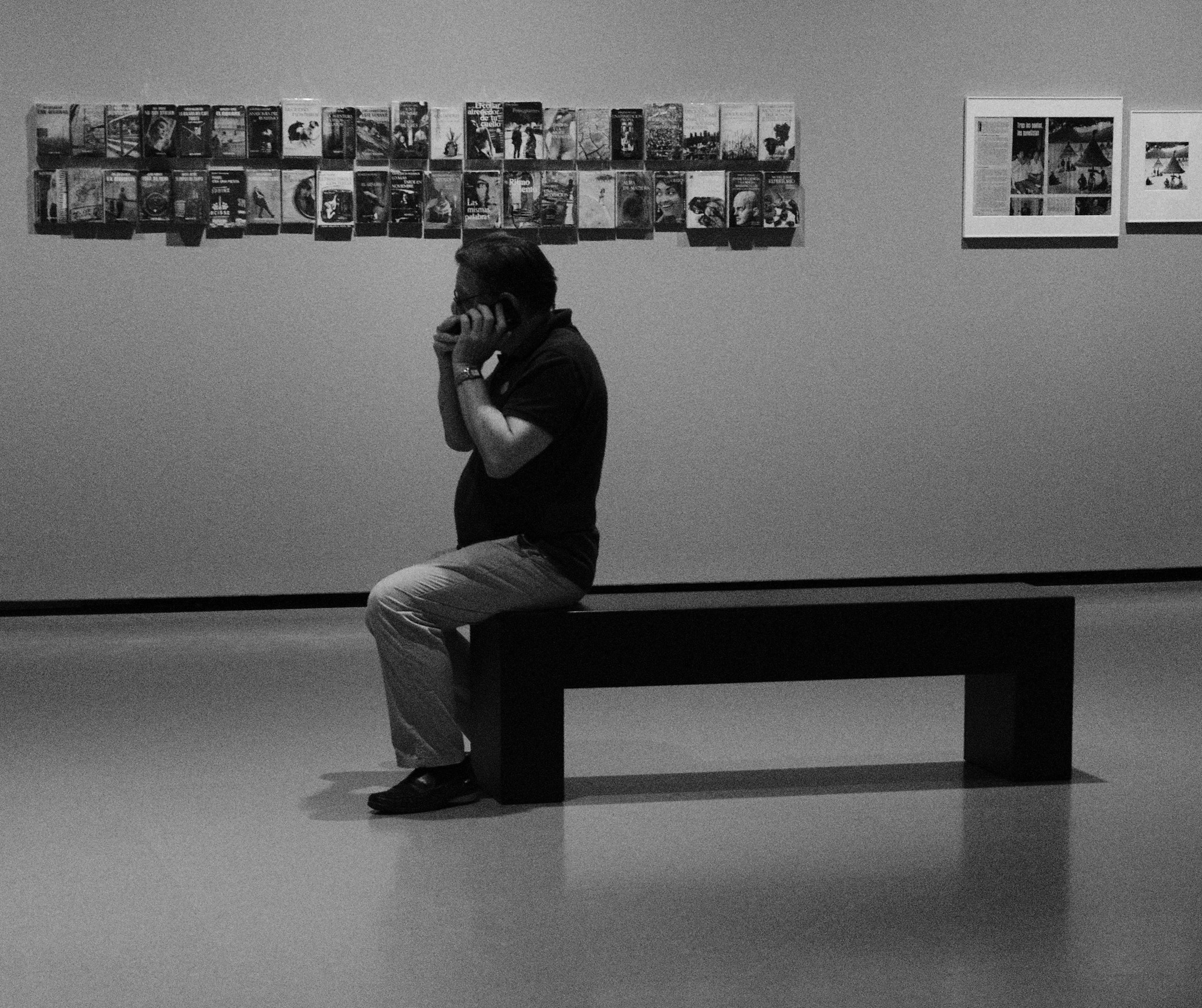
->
<box><xmin>452</xmin><ymin>363</ymin><xmax>483</xmax><ymax>387</ymax></box>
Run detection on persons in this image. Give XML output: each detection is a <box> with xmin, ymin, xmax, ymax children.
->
<box><xmin>35</xmin><ymin>105</ymin><xmax>799</xmax><ymax>229</ymax></box>
<box><xmin>1012</xmin><ymin>118</ymin><xmax>1114</xmax><ymax>216</ymax></box>
<box><xmin>367</xmin><ymin>232</ymin><xmax>609</xmax><ymax>815</ymax></box>
<box><xmin>1145</xmin><ymin>145</ymin><xmax>1190</xmax><ymax>190</ymax></box>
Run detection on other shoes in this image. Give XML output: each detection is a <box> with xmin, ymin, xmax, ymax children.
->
<box><xmin>461</xmin><ymin>751</ymin><xmax>501</xmax><ymax>798</ymax></box>
<box><xmin>367</xmin><ymin>764</ymin><xmax>481</xmax><ymax>814</ymax></box>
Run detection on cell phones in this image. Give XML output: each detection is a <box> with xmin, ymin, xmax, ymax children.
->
<box><xmin>449</xmin><ymin>297</ymin><xmax>522</xmax><ymax>336</ymax></box>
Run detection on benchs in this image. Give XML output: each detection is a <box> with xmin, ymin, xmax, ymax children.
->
<box><xmin>470</xmin><ymin>569</ymin><xmax>1079</xmax><ymax>804</ymax></box>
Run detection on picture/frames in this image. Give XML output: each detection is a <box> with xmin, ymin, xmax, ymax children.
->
<box><xmin>1128</xmin><ymin>109</ymin><xmax>1202</xmax><ymax>223</ymax></box>
<box><xmin>962</xmin><ymin>95</ymin><xmax>1126</xmax><ymax>242</ymax></box>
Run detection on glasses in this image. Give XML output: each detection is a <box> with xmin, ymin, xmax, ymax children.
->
<box><xmin>453</xmin><ymin>289</ymin><xmax>482</xmax><ymax>307</ymax></box>
